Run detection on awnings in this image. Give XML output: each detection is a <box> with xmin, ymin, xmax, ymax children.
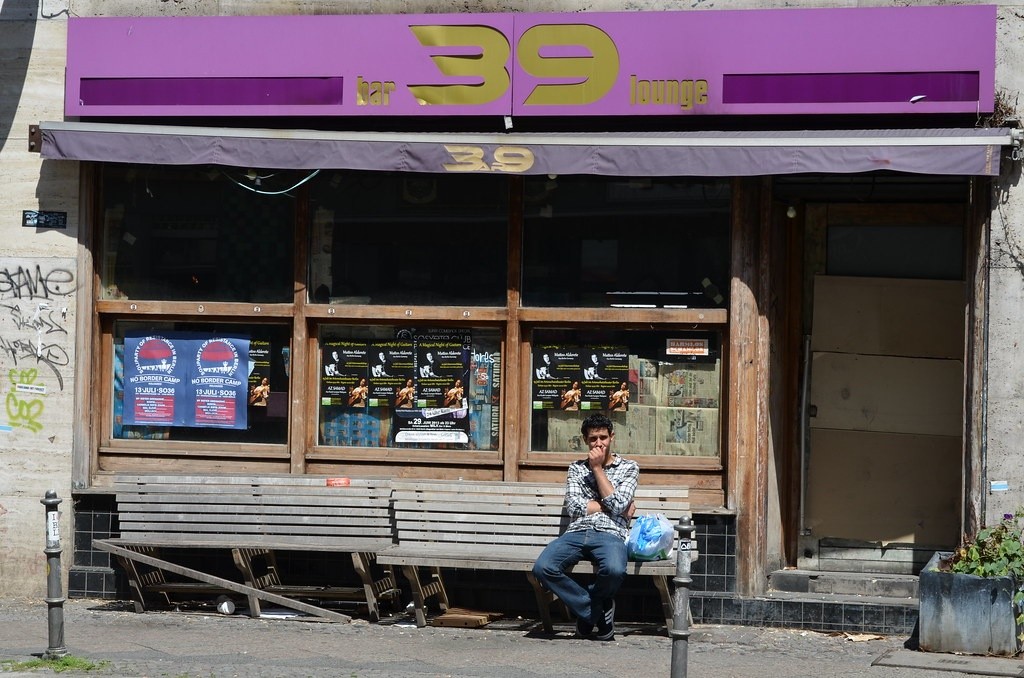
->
<box><xmin>39</xmin><ymin>121</ymin><xmax>1013</xmax><ymax>178</ymax></box>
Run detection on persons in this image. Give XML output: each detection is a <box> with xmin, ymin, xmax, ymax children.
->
<box><xmin>397</xmin><ymin>379</ymin><xmax>414</xmax><ymax>409</ymax></box>
<box><xmin>445</xmin><ymin>380</ymin><xmax>463</xmax><ymax>407</ymax></box>
<box><xmin>325</xmin><ymin>350</ymin><xmax>346</xmax><ymax>376</ymax></box>
<box><xmin>613</xmin><ymin>382</ymin><xmax>628</xmax><ymax>411</ymax></box>
<box><xmin>536</xmin><ymin>352</ymin><xmax>557</xmax><ymax>379</ymax></box>
<box><xmin>349</xmin><ymin>378</ymin><xmax>368</xmax><ymax>407</ymax></box>
<box><xmin>253</xmin><ymin>377</ymin><xmax>269</xmax><ymax>406</ymax></box>
<box><xmin>420</xmin><ymin>351</ymin><xmax>440</xmax><ymax>377</ymax></box>
<box><xmin>371</xmin><ymin>351</ymin><xmax>393</xmax><ymax>377</ymax></box>
<box><xmin>584</xmin><ymin>353</ymin><xmax>606</xmax><ymax>379</ymax></box>
<box><xmin>532</xmin><ymin>412</ymin><xmax>640</xmax><ymax>638</ymax></box>
<box><xmin>564</xmin><ymin>381</ymin><xmax>581</xmax><ymax>411</ymax></box>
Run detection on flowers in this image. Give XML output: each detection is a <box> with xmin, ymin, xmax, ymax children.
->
<box><xmin>950</xmin><ymin>506</ymin><xmax>1024</xmax><ymax>644</ymax></box>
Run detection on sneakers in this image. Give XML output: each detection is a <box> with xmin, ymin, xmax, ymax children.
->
<box><xmin>576</xmin><ymin>616</ymin><xmax>594</xmax><ymax>636</ymax></box>
<box><xmin>596</xmin><ymin>598</ymin><xmax>615</xmax><ymax>641</ymax></box>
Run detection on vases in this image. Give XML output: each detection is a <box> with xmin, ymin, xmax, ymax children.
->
<box><xmin>918</xmin><ymin>551</ymin><xmax>1024</xmax><ymax>657</ymax></box>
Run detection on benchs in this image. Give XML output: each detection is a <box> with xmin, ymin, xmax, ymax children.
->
<box><xmin>90</xmin><ymin>473</ymin><xmax>698</xmax><ymax>639</ymax></box>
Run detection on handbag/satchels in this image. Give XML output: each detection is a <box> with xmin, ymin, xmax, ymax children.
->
<box><xmin>624</xmin><ymin>511</ymin><xmax>674</xmax><ymax>560</ymax></box>
